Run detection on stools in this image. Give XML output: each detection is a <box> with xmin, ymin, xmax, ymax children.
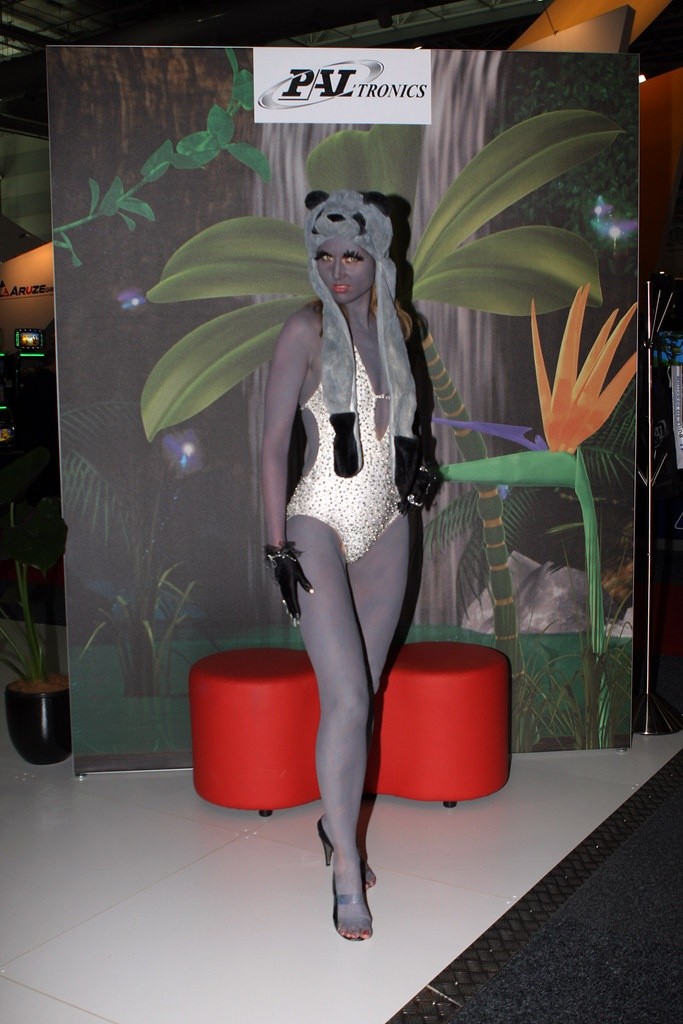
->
<box><xmin>189</xmin><ymin>649</ymin><xmax>322</xmax><ymax>818</ymax></box>
<box><xmin>364</xmin><ymin>642</ymin><xmax>511</xmax><ymax>807</ymax></box>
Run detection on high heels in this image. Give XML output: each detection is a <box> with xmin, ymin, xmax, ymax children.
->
<box><xmin>333</xmin><ymin>869</ymin><xmax>372</xmax><ymax>941</ymax></box>
<box><xmin>317</xmin><ymin>816</ymin><xmax>376</xmax><ymax>891</ymax></box>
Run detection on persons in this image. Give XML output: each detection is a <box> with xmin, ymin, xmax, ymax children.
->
<box><xmin>262</xmin><ymin>188</ymin><xmax>438</xmax><ymax>945</ymax></box>
<box><xmin>13</xmin><ymin>318</ymin><xmax>61</xmax><ymax>510</ymax></box>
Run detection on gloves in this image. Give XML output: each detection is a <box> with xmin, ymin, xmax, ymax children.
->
<box><xmin>397</xmin><ymin>466</ymin><xmax>441</xmax><ymax>517</ymax></box>
<box><xmin>263</xmin><ymin>540</ymin><xmax>314</xmax><ymax>627</ymax></box>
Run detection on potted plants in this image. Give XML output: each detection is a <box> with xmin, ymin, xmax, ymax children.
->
<box><xmin>0</xmin><ymin>445</ymin><xmax>72</xmax><ymax>765</ymax></box>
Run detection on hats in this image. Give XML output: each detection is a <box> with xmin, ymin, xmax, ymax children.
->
<box><xmin>304</xmin><ymin>191</ymin><xmax>419</xmax><ymax>497</ymax></box>
